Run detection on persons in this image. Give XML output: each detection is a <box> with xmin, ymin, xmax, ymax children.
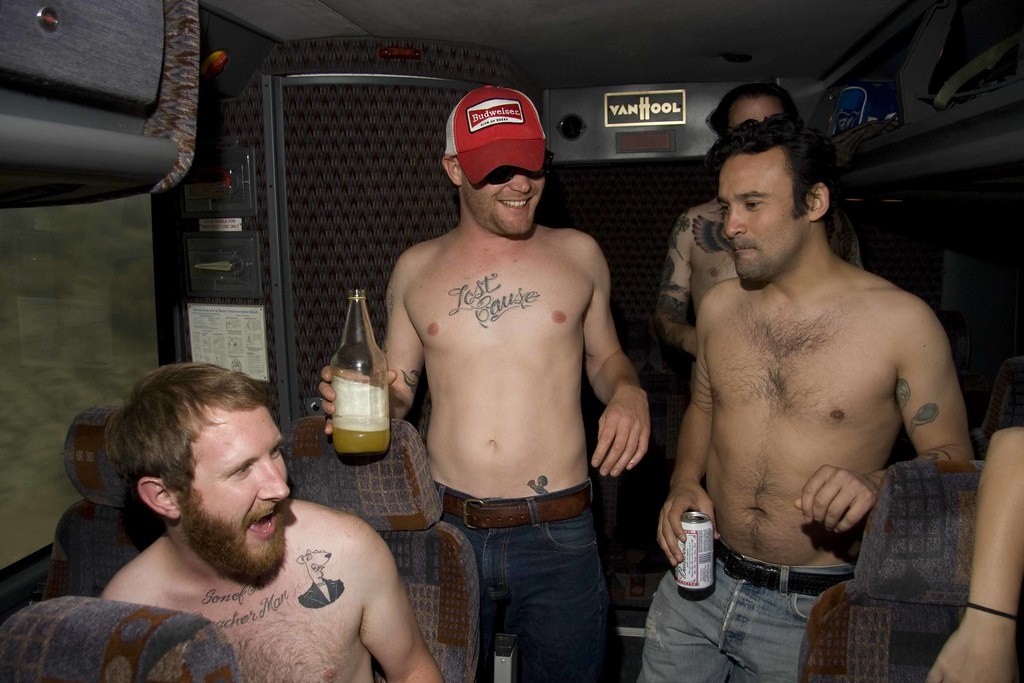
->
<box><xmin>637</xmin><ymin>112</ymin><xmax>972</xmax><ymax>683</ymax></box>
<box><xmin>655</xmin><ymin>82</ymin><xmax>863</xmax><ymax>356</ymax></box>
<box><xmin>101</xmin><ymin>365</ymin><xmax>442</xmax><ymax>683</ymax></box>
<box><xmin>318</xmin><ymin>85</ymin><xmax>650</xmax><ymax>683</ymax></box>
<box><xmin>923</xmin><ymin>358</ymin><xmax>1024</xmax><ymax>683</ymax></box>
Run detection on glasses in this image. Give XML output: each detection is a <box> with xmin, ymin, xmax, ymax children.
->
<box><xmin>487</xmin><ymin>147</ymin><xmax>554</xmax><ymax>185</ymax></box>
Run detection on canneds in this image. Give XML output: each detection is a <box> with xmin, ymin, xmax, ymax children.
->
<box><xmin>673</xmin><ymin>512</ymin><xmax>714</xmax><ymax>590</ymax></box>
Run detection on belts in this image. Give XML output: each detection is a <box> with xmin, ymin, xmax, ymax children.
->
<box><xmin>436</xmin><ymin>486</ymin><xmax>591</xmax><ymax>530</ymax></box>
<box><xmin>714</xmin><ymin>537</ymin><xmax>855</xmax><ymax>597</ymax></box>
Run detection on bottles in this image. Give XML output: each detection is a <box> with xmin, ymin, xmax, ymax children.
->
<box><xmin>330</xmin><ymin>289</ymin><xmax>391</xmax><ymax>453</ymax></box>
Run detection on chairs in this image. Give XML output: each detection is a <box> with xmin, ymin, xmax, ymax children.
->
<box><xmin>0</xmin><ymin>593</ymin><xmax>242</xmax><ymax>683</ymax></box>
<box><xmin>936</xmin><ymin>301</ymin><xmax>995</xmax><ymax>431</ymax></box>
<box><xmin>42</xmin><ymin>405</ymin><xmax>170</xmax><ymax>600</ymax></box>
<box><xmin>796</xmin><ymin>458</ymin><xmax>986</xmax><ymax>683</ymax></box>
<box><xmin>278</xmin><ymin>414</ymin><xmax>482</xmax><ymax>683</ymax></box>
<box><xmin>967</xmin><ymin>356</ymin><xmax>1024</xmax><ymax>462</ymax></box>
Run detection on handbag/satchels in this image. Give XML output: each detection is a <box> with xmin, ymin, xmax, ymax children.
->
<box><xmin>832</xmin><ymin>77</ymin><xmax>899</xmax><ymax>136</ymax></box>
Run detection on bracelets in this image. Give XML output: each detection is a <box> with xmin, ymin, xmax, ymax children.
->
<box><xmin>966</xmin><ymin>602</ymin><xmax>1017</xmax><ymax>621</ymax></box>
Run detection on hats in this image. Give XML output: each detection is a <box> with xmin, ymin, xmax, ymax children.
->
<box><xmin>444</xmin><ymin>85</ymin><xmax>546</xmax><ymax>185</ymax></box>
